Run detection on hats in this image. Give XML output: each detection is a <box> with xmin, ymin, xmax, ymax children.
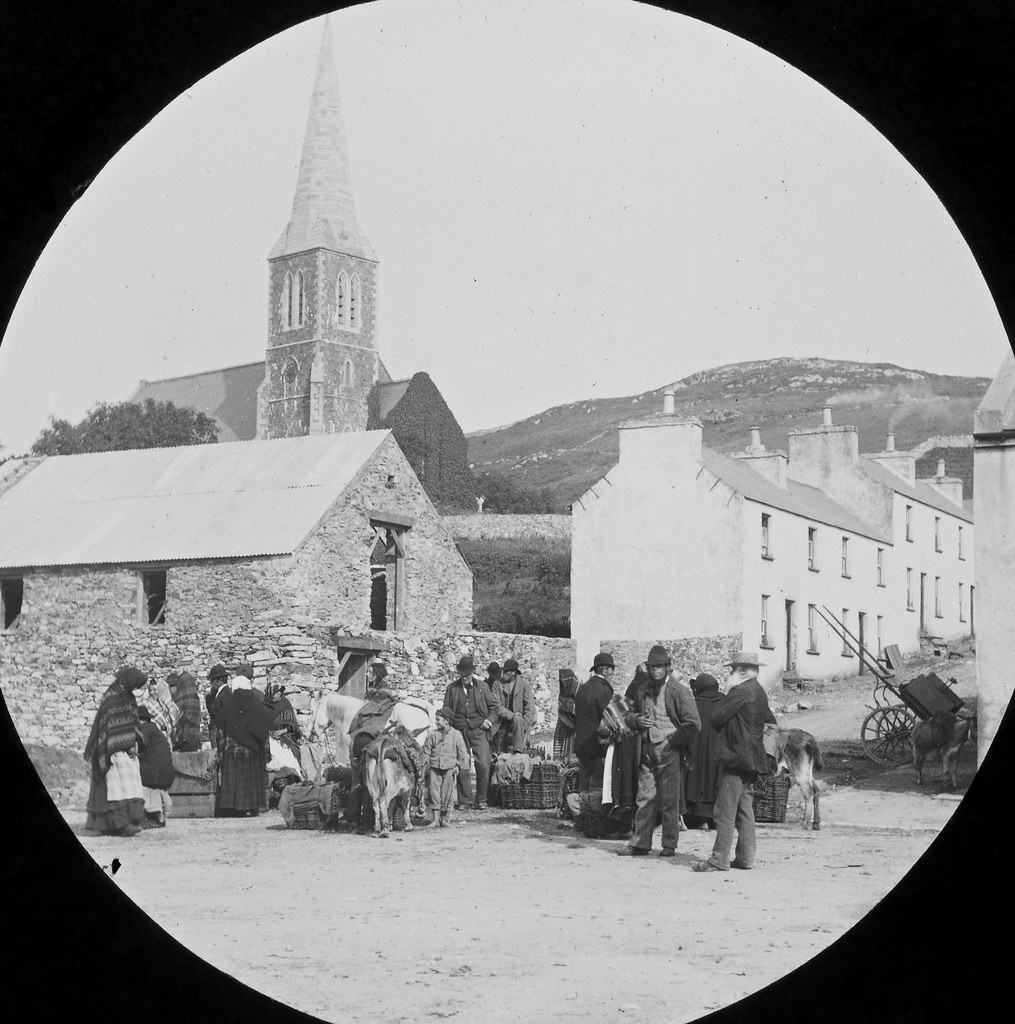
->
<box><xmin>236</xmin><ymin>665</ymin><xmax>253</xmax><ymax>678</ymax></box>
<box><xmin>487</xmin><ymin>663</ymin><xmax>503</xmax><ymax>673</ymax></box>
<box><xmin>589</xmin><ymin>653</ymin><xmax>620</xmax><ymax>673</ymax></box>
<box><xmin>207</xmin><ymin>664</ymin><xmax>230</xmax><ymax>679</ymax></box>
<box><xmin>137</xmin><ymin>705</ymin><xmax>154</xmax><ymax>718</ymax></box>
<box><xmin>456</xmin><ymin>657</ymin><xmax>474</xmax><ymax>676</ymax></box>
<box><xmin>644</xmin><ymin>645</ymin><xmax>670</xmax><ymax>667</ymax></box>
<box><xmin>500</xmin><ymin>660</ymin><xmax>521</xmax><ymax>674</ymax></box>
<box><xmin>724</xmin><ymin>651</ymin><xmax>766</xmax><ymax>667</ymax></box>
<box><xmin>436</xmin><ymin>706</ymin><xmax>453</xmax><ymax>720</ymax></box>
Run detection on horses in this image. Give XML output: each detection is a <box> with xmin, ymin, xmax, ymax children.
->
<box><xmin>306</xmin><ymin>690</ymin><xmax>436</xmax><ymax>770</ymax></box>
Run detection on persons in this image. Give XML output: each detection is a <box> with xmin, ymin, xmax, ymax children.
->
<box><xmin>82</xmin><ymin>662</ymin><xmax>306</xmax><ymax>836</ymax></box>
<box><xmin>480</xmin><ymin>661</ymin><xmax>503</xmax><ymax>692</ymax></box>
<box><xmin>441</xmin><ymin>657</ymin><xmax>498</xmax><ymax>810</ymax></box>
<box><xmin>421</xmin><ymin>707</ymin><xmax>468</xmax><ymax>827</ymax></box>
<box><xmin>364</xmin><ymin>663</ymin><xmax>393</xmax><ymax>702</ymax></box>
<box><xmin>692</xmin><ymin>651</ymin><xmax>768</xmax><ymax>872</ymax></box>
<box><xmin>574</xmin><ymin>652</ymin><xmax>618</xmax><ymax>838</ymax></box>
<box><xmin>492</xmin><ymin>659</ymin><xmax>536</xmax><ymax>759</ymax></box>
<box><xmin>615</xmin><ymin>645</ymin><xmax>702</xmax><ymax>856</ymax></box>
<box><xmin>623</xmin><ymin>661</ymin><xmax>726</xmax><ymax>831</ymax></box>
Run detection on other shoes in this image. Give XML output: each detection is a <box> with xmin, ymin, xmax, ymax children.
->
<box><xmin>731</xmin><ymin>859</ymin><xmax>752</xmax><ymax>868</ymax></box>
<box><xmin>477</xmin><ymin>802</ymin><xmax>487</xmax><ymax>810</ymax></box>
<box><xmin>118</xmin><ymin>823</ymin><xmax>142</xmax><ymax>836</ymax></box>
<box><xmin>692</xmin><ymin>861</ymin><xmax>718</xmax><ymax>871</ymax></box>
<box><xmin>661</xmin><ymin>845</ymin><xmax>674</xmax><ymax>856</ymax></box>
<box><xmin>146</xmin><ymin>818</ymin><xmax>165</xmax><ymax>827</ymax></box>
<box><xmin>427</xmin><ymin>822</ymin><xmax>441</xmax><ymax>829</ymax></box>
<box><xmin>442</xmin><ymin>820</ymin><xmax>451</xmax><ymax>827</ymax></box>
<box><xmin>615</xmin><ymin>844</ymin><xmax>648</xmax><ymax>855</ymax></box>
<box><xmin>455</xmin><ymin>802</ymin><xmax>471</xmax><ymax>809</ymax></box>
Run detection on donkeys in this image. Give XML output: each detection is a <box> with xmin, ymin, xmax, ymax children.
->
<box><xmin>765</xmin><ymin>723</ymin><xmax>825</xmax><ymax>831</ymax></box>
<box><xmin>911</xmin><ymin>712</ymin><xmax>979</xmax><ymax>789</ymax></box>
<box><xmin>364</xmin><ymin>724</ymin><xmax>431</xmax><ymax>838</ymax></box>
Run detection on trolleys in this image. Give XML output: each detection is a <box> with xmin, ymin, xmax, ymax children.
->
<box><xmin>811</xmin><ymin>605</ymin><xmax>966</xmax><ymax>768</ymax></box>
